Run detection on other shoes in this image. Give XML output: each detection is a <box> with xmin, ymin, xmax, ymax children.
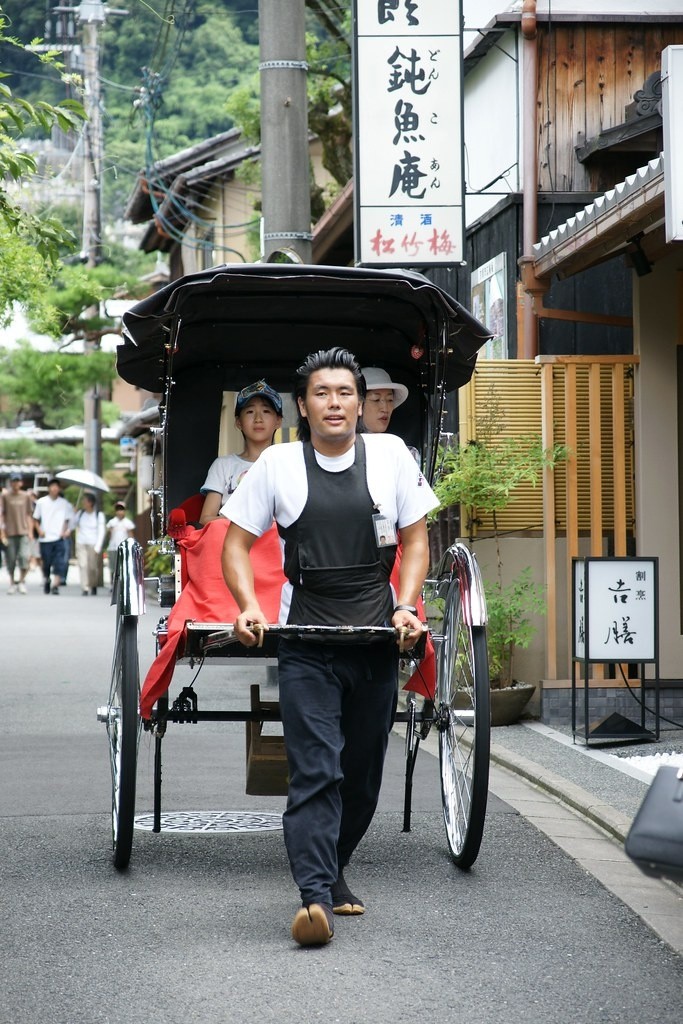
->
<box><xmin>83</xmin><ymin>590</ymin><xmax>87</xmax><ymax>595</ymax></box>
<box><xmin>327</xmin><ymin>866</ymin><xmax>365</xmax><ymax>914</ymax></box>
<box><xmin>92</xmin><ymin>586</ymin><xmax>96</xmax><ymax>595</ymax></box>
<box><xmin>291</xmin><ymin>902</ymin><xmax>335</xmax><ymax>945</ymax></box>
<box><xmin>44</xmin><ymin>579</ymin><xmax>51</xmax><ymax>594</ymax></box>
<box><xmin>52</xmin><ymin>586</ymin><xmax>59</xmax><ymax>595</ymax></box>
<box><xmin>7</xmin><ymin>586</ymin><xmax>15</xmax><ymax>594</ymax></box>
<box><xmin>18</xmin><ymin>582</ymin><xmax>25</xmax><ymax>593</ymax></box>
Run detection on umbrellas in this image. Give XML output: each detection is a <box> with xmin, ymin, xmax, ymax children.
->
<box><xmin>55</xmin><ymin>469</ymin><xmax>109</xmax><ymax>506</ymax></box>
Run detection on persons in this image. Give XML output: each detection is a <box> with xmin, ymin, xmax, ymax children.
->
<box><xmin>74</xmin><ymin>493</ymin><xmax>105</xmax><ymax>596</ymax></box>
<box><xmin>361</xmin><ymin>367</ymin><xmax>408</xmax><ymax>433</ymax></box>
<box><xmin>199</xmin><ymin>380</ymin><xmax>283</xmax><ymax>525</ymax></box>
<box><xmin>106</xmin><ymin>501</ymin><xmax>136</xmax><ymax>592</ymax></box>
<box><xmin>0</xmin><ymin>473</ymin><xmax>36</xmax><ymax>594</ymax></box>
<box><xmin>58</xmin><ymin>490</ymin><xmax>71</xmax><ymax>585</ymax></box>
<box><xmin>32</xmin><ymin>479</ymin><xmax>73</xmax><ymax>593</ymax></box>
<box><xmin>219</xmin><ymin>346</ymin><xmax>441</xmax><ymax>944</ymax></box>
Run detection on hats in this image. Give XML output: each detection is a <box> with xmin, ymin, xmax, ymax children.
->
<box><xmin>360</xmin><ymin>367</ymin><xmax>408</xmax><ymax>408</ymax></box>
<box><xmin>10</xmin><ymin>472</ymin><xmax>22</xmax><ymax>480</ymax></box>
<box><xmin>236</xmin><ymin>380</ymin><xmax>281</xmax><ymax>412</ymax></box>
<box><xmin>115</xmin><ymin>500</ymin><xmax>126</xmax><ymax>507</ymax></box>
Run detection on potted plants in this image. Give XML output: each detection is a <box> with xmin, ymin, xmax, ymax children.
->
<box><xmin>449</xmin><ymin>564</ymin><xmax>549</xmax><ymax>726</ymax></box>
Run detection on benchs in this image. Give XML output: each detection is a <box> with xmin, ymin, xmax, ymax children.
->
<box><xmin>168</xmin><ymin>495</ymin><xmax>201</xmax><ymax>603</ymax></box>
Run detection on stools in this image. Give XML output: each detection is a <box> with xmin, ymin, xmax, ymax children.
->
<box><xmin>246</xmin><ymin>684</ymin><xmax>290</xmax><ymax>796</ymax></box>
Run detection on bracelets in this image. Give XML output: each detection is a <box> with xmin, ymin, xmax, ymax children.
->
<box><xmin>395</xmin><ymin>605</ymin><xmax>418</xmax><ymax>617</ymax></box>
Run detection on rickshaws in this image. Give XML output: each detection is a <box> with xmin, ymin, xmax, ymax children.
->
<box><xmin>97</xmin><ymin>260</ymin><xmax>494</xmax><ymax>871</ymax></box>
